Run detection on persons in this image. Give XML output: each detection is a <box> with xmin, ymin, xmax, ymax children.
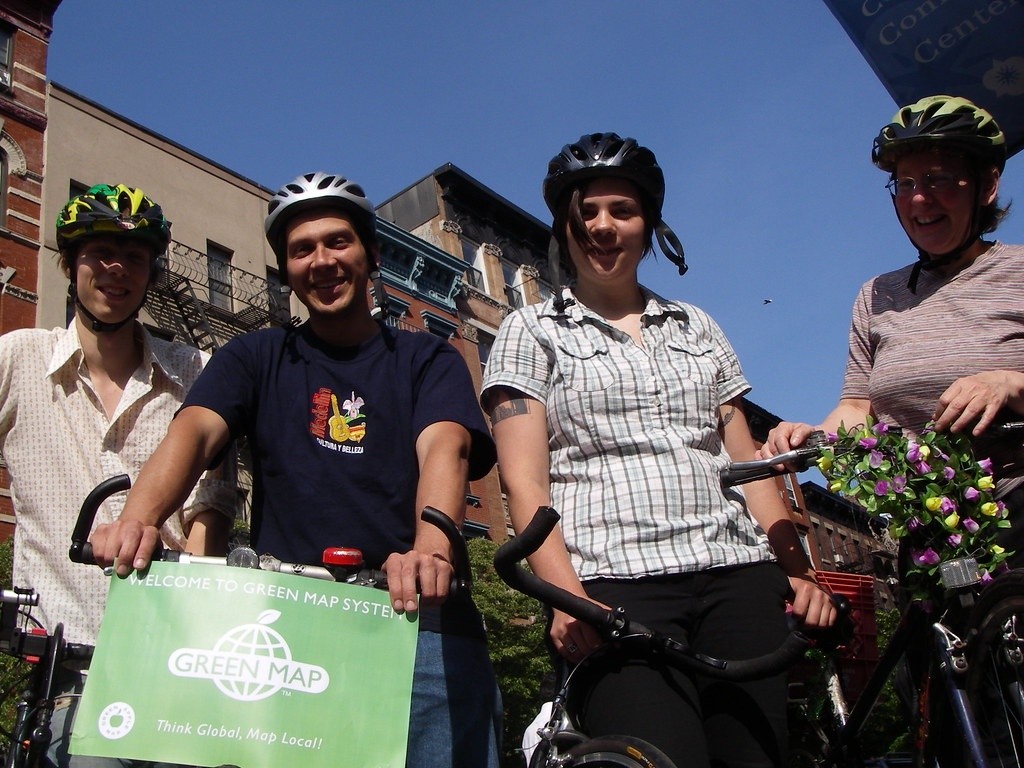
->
<box><xmin>0</xmin><ymin>184</ymin><xmax>240</xmax><ymax>768</ymax></box>
<box><xmin>755</xmin><ymin>94</ymin><xmax>1024</xmax><ymax>609</ymax></box>
<box><xmin>479</xmin><ymin>132</ymin><xmax>838</xmax><ymax>768</ymax></box>
<box><xmin>89</xmin><ymin>169</ymin><xmax>496</xmax><ymax>768</ymax></box>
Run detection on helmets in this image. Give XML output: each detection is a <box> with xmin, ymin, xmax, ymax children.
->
<box><xmin>872</xmin><ymin>95</ymin><xmax>1007</xmax><ymax>174</ymax></box>
<box><xmin>543</xmin><ymin>132</ymin><xmax>688</xmax><ymax>225</ymax></box>
<box><xmin>55</xmin><ymin>183</ymin><xmax>172</xmax><ymax>254</ymax></box>
<box><xmin>265</xmin><ymin>171</ymin><xmax>389</xmax><ymax>254</ymax></box>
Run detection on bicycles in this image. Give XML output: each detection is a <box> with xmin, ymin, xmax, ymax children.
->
<box><xmin>719</xmin><ymin>419</ymin><xmax>1024</xmax><ymax>768</ymax></box>
<box><xmin>0</xmin><ymin>579</ymin><xmax>99</xmax><ymax>768</ymax></box>
<box><xmin>492</xmin><ymin>504</ymin><xmax>851</xmax><ymax>767</ymax></box>
<box><xmin>66</xmin><ymin>470</ymin><xmax>471</xmax><ymax>614</ymax></box>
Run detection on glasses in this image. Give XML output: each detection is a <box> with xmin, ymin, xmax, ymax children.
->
<box><xmin>885</xmin><ymin>171</ymin><xmax>969</xmax><ymax>197</ymax></box>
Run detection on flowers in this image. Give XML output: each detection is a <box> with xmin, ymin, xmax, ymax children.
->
<box><xmin>817</xmin><ymin>415</ymin><xmax>1015</xmax><ymax>586</ymax></box>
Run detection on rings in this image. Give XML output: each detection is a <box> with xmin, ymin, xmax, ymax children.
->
<box><xmin>567</xmin><ymin>642</ymin><xmax>579</xmax><ymax>654</ymax></box>
